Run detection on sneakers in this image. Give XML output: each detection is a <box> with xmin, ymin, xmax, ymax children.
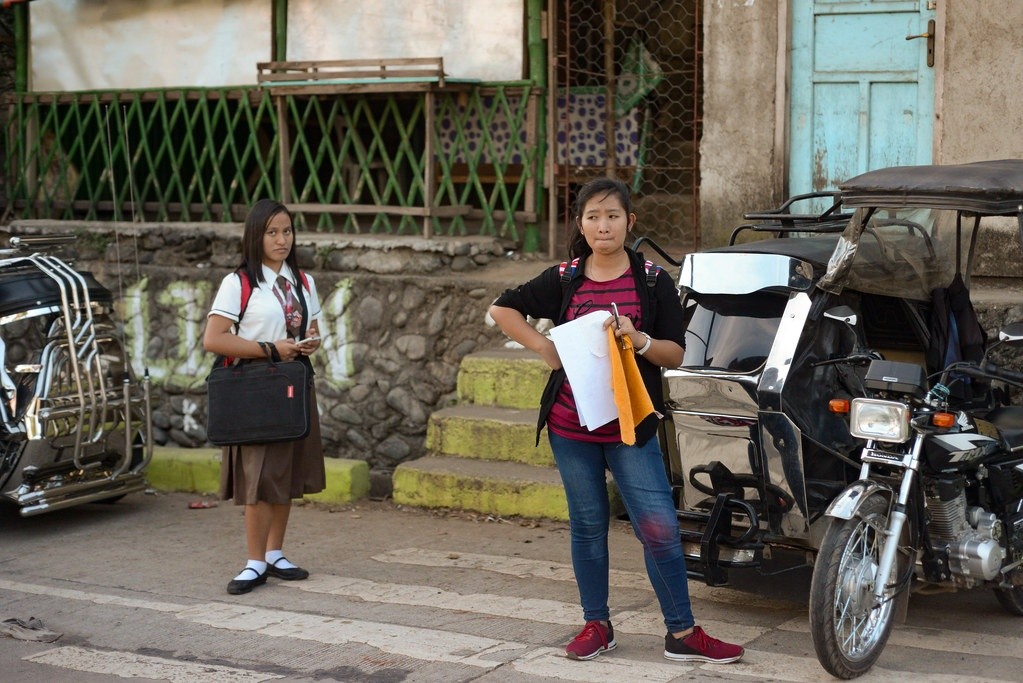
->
<box><xmin>565</xmin><ymin>619</ymin><xmax>618</xmax><ymax>661</ymax></box>
<box><xmin>664</xmin><ymin>625</ymin><xmax>745</xmax><ymax>664</ymax></box>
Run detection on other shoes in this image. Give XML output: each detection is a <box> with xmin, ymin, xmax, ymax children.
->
<box><xmin>266</xmin><ymin>557</ymin><xmax>309</xmax><ymax>580</ymax></box>
<box><xmin>227</xmin><ymin>568</ymin><xmax>267</xmax><ymax>595</ymax></box>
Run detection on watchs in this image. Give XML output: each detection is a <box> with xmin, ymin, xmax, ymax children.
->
<box><xmin>635</xmin><ymin>331</ymin><xmax>651</xmax><ymax>355</ymax></box>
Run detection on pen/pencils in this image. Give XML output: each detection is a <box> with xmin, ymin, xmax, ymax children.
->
<box><xmin>611</xmin><ymin>302</ymin><xmax>624</xmax><ymax>350</ymax></box>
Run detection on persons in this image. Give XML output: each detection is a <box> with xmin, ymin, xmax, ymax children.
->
<box><xmin>205</xmin><ymin>201</ymin><xmax>326</xmax><ymax>595</ymax></box>
<box><xmin>489</xmin><ymin>179</ymin><xmax>747</xmax><ymax>665</ymax></box>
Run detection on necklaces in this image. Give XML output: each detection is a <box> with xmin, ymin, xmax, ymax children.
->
<box><xmin>591</xmin><ymin>253</ymin><xmax>629</xmax><ymax>280</ymax></box>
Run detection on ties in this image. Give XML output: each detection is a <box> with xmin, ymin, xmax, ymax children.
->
<box><xmin>276</xmin><ymin>274</ymin><xmax>304</xmax><ymax>338</ymax></box>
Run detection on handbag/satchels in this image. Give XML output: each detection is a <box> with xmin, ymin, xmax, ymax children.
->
<box><xmin>206</xmin><ymin>341</ymin><xmax>312</xmax><ymax>446</ymax></box>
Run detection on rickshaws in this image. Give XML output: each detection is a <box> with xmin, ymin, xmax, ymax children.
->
<box><xmin>658</xmin><ymin>158</ymin><xmax>1022</xmax><ymax>683</ymax></box>
<box><xmin>1</xmin><ymin>231</ymin><xmax>154</xmax><ymax>520</ymax></box>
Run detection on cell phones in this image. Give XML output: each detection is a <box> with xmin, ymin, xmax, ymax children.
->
<box><xmin>294</xmin><ymin>337</ymin><xmax>322</xmax><ymax>345</ymax></box>
